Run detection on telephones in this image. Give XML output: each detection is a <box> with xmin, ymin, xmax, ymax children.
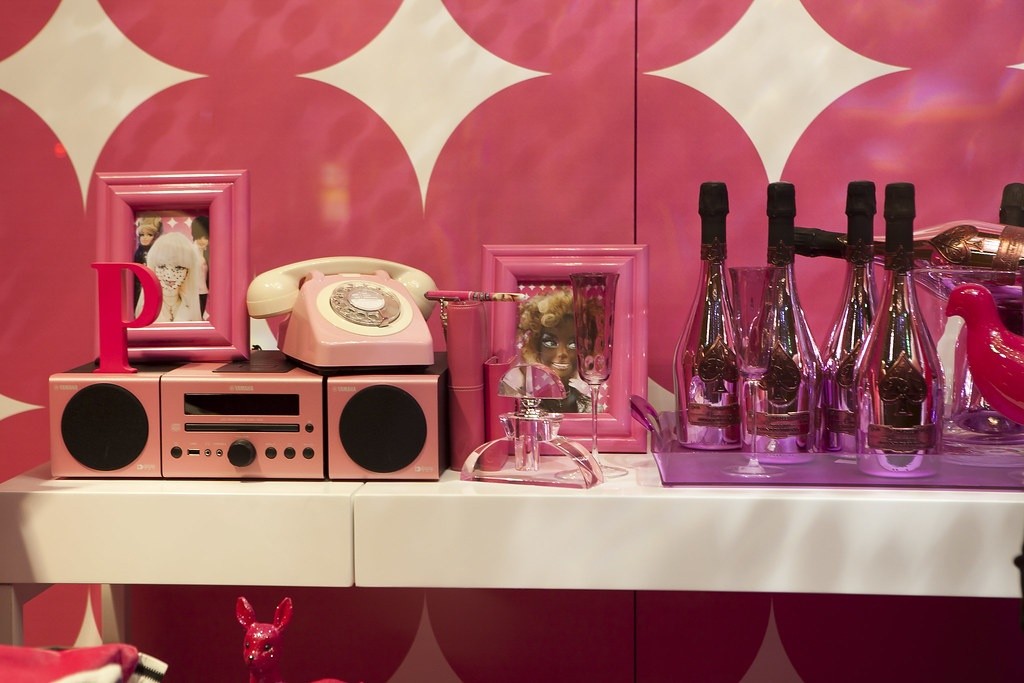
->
<box><xmin>246</xmin><ymin>256</ymin><xmax>439</xmax><ymax>374</ymax></box>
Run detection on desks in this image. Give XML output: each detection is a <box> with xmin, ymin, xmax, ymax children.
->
<box><xmin>1</xmin><ymin>436</ymin><xmax>1024</xmax><ymax>683</ymax></box>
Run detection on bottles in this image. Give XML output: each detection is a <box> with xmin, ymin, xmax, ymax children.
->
<box><xmin>674</xmin><ymin>182</ymin><xmax>1024</xmax><ymax>479</ymax></box>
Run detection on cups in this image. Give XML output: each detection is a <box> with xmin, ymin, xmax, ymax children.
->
<box><xmin>910</xmin><ymin>268</ymin><xmax>1024</xmax><ymax>468</ymax></box>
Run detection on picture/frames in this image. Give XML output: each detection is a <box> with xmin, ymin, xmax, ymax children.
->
<box><xmin>93</xmin><ymin>165</ymin><xmax>250</xmax><ymax>357</ymax></box>
<box><xmin>478</xmin><ymin>240</ymin><xmax>654</xmax><ymax>453</ymax></box>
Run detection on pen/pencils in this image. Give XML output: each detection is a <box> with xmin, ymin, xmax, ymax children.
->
<box><xmin>424</xmin><ymin>291</ymin><xmax>530</xmax><ymax>302</ymax></box>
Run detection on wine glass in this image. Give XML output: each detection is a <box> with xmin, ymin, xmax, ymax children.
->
<box><xmin>553</xmin><ymin>272</ymin><xmax>628</xmax><ymax>479</ymax></box>
